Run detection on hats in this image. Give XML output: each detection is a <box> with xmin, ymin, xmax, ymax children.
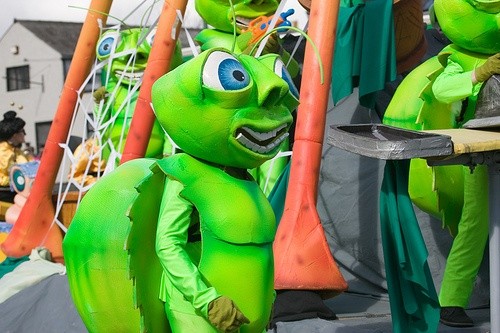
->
<box><xmin>0</xmin><ymin>117</ymin><xmax>25</xmax><ymax>140</ymax></box>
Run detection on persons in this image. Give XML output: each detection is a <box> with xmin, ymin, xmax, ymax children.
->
<box><xmin>63</xmin><ymin>0</ymin><xmax>325</xmax><ymax>333</ymax></box>
<box><xmin>0</xmin><ymin>117</ymin><xmax>29</xmax><ymax>222</ymax></box>
<box><xmin>382</xmin><ymin>0</ymin><xmax>500</xmax><ymax>328</ymax></box>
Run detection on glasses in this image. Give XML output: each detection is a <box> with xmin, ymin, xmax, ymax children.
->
<box><xmin>17</xmin><ymin>129</ymin><xmax>26</xmax><ymax>135</ymax></box>
